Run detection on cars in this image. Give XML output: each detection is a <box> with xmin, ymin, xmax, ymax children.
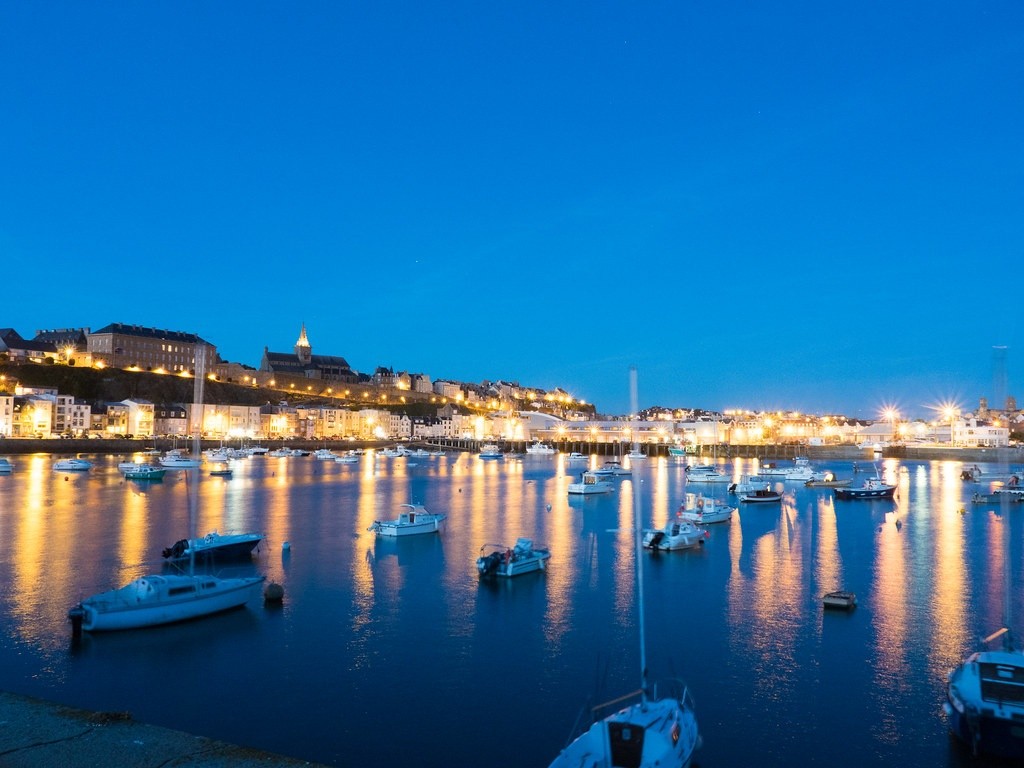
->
<box><xmin>51</xmin><ymin>431</ymin><xmax>102</xmax><ymax>440</ymax></box>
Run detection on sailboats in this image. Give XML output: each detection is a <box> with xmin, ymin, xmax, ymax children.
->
<box><xmin>541</xmin><ymin>368</ymin><xmax>705</xmax><ymax>768</ymax></box>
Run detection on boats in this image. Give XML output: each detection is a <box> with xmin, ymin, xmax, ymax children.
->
<box><xmin>368</xmin><ymin>484</ymin><xmax>450</xmax><ymax>540</ymax></box>
<box><xmin>160</xmin><ymin>470</ymin><xmax>264</xmax><ymax>573</ymax></box>
<box><xmin>477</xmin><ymin>443</ymin><xmax>503</xmax><ymax>460</ymax></box>
<box><xmin>676</xmin><ymin>491</ymin><xmax>738</xmax><ymax>524</ymax></box>
<box><xmin>202</xmin><ymin>436</ymin><xmax>448</xmax><ymax>464</ymax></box>
<box><xmin>476</xmin><ymin>538</ymin><xmax>553</xmax><ymax>583</ymax></box>
<box><xmin>113</xmin><ymin>429</ymin><xmax>204</xmax><ymax>480</ymax></box>
<box><xmin>566</xmin><ymin>449</ymin><xmax>590</xmax><ymax>462</ymax></box>
<box><xmin>821</xmin><ymin>589</ymin><xmax>861</xmax><ymax>612</ymax></box>
<box><xmin>961</xmin><ymin>462</ymin><xmax>1024</xmax><ymax>509</ymax></box>
<box><xmin>626</xmin><ymin>449</ymin><xmax>648</xmax><ymax>459</ymax></box>
<box><xmin>668</xmin><ymin>444</ymin><xmax>834</xmax><ymax>504</ymax></box>
<box><xmin>833</xmin><ymin>461</ymin><xmax>899</xmax><ymax>500</ymax></box>
<box><xmin>640</xmin><ymin>517</ymin><xmax>713</xmax><ymax>554</ymax></box>
<box><xmin>852</xmin><ymin>461</ymin><xmax>888</xmax><ymax>474</ymax></box>
<box><xmin>53</xmin><ymin>458</ymin><xmax>94</xmax><ymax>472</ymax></box>
<box><xmin>210</xmin><ymin>469</ymin><xmax>233</xmax><ymax>477</ymax></box>
<box><xmin>524</xmin><ymin>438</ymin><xmax>560</xmax><ymax>457</ymax></box>
<box><xmin>0</xmin><ymin>459</ymin><xmax>17</xmax><ymax>476</ymax></box>
<box><xmin>503</xmin><ymin>449</ymin><xmax>526</xmax><ymax>460</ymax></box>
<box><xmin>941</xmin><ymin>631</ymin><xmax>1024</xmax><ymax>765</ymax></box>
<box><xmin>71</xmin><ymin>476</ymin><xmax>268</xmax><ymax>633</ymax></box>
<box><xmin>563</xmin><ymin>458</ymin><xmax>633</xmax><ymax>496</ymax></box>
